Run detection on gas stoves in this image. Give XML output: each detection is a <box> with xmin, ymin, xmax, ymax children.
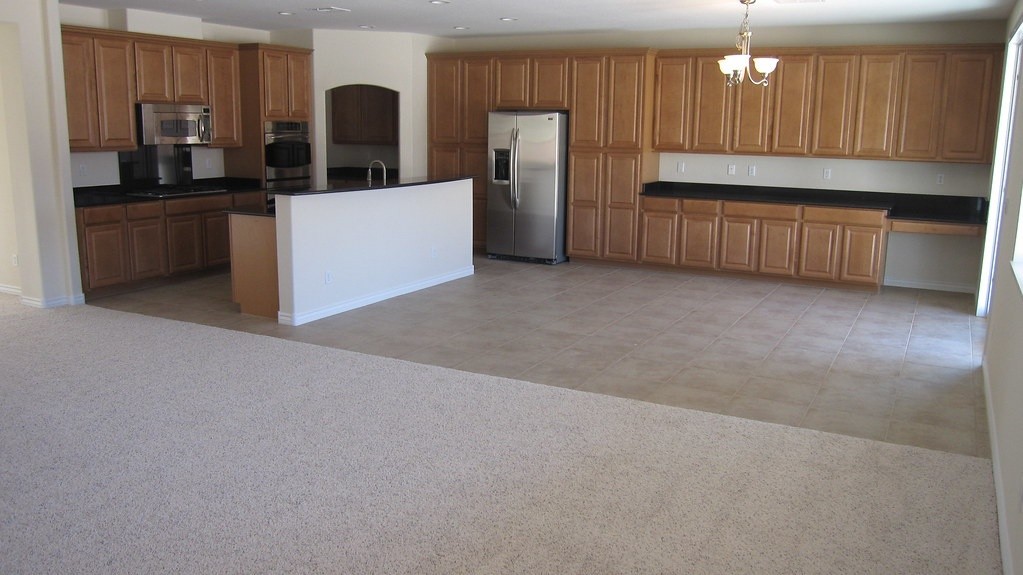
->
<box><xmin>127</xmin><ymin>185</ymin><xmax>227</xmax><ymax>196</ymax></box>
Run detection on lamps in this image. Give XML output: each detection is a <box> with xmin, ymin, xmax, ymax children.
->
<box><xmin>718</xmin><ymin>0</ymin><xmax>779</xmax><ymax>87</ymax></box>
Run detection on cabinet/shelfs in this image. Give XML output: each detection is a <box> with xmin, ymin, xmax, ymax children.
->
<box><xmin>653</xmin><ymin>42</ymin><xmax>1005</xmax><ymax>165</ymax></box>
<box><xmin>227</xmin><ymin>214</ymin><xmax>280</xmax><ymax>320</ymax></box>
<box><xmin>75</xmin><ymin>200</ymin><xmax>169</xmax><ymax>300</ymax></box>
<box><xmin>222</xmin><ymin>43</ymin><xmax>314</xmax><ymax>189</ymax></box>
<box><xmin>424</xmin><ymin>51</ymin><xmax>492</xmax><ymax>254</ymax></box>
<box><xmin>61</xmin><ymin>24</ymin><xmax>138</xmax><ymax>152</ymax></box>
<box><xmin>133</xmin><ymin>32</ymin><xmax>209</xmax><ymax>105</ymax></box>
<box><xmin>331</xmin><ymin>85</ymin><xmax>399</xmax><ymax>146</ymax></box>
<box><xmin>165</xmin><ymin>193</ymin><xmax>233</xmax><ymax>285</ymax></box>
<box><xmin>638</xmin><ymin>196</ymin><xmax>888</xmax><ymax>292</ymax></box>
<box><xmin>566</xmin><ymin>48</ymin><xmax>659</xmax><ymax>269</ymax></box>
<box><xmin>233</xmin><ymin>190</ymin><xmax>266</xmax><ymax>214</ymax></box>
<box><xmin>207</xmin><ymin>40</ymin><xmax>242</xmax><ymax>148</ymax></box>
<box><xmin>493</xmin><ymin>49</ymin><xmax>570</xmax><ymax>110</ymax></box>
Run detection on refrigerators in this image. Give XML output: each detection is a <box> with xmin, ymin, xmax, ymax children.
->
<box><xmin>487</xmin><ymin>109</ymin><xmax>569</xmax><ymax>265</ymax></box>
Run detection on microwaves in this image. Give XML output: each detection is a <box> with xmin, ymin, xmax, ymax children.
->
<box><xmin>136</xmin><ymin>103</ymin><xmax>209</xmax><ymax>144</ymax></box>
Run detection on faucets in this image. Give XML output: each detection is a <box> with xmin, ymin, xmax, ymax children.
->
<box><xmin>367</xmin><ymin>159</ymin><xmax>388</xmax><ymax>188</ymax></box>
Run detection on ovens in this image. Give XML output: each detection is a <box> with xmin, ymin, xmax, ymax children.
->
<box><xmin>264</xmin><ymin>121</ymin><xmax>313</xmax><ymax>187</ymax></box>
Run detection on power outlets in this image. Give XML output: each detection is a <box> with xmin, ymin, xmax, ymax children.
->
<box><xmin>748</xmin><ymin>165</ymin><xmax>755</xmax><ymax>176</ymax></box>
<box><xmin>823</xmin><ymin>168</ymin><xmax>830</xmax><ymax>179</ymax></box>
